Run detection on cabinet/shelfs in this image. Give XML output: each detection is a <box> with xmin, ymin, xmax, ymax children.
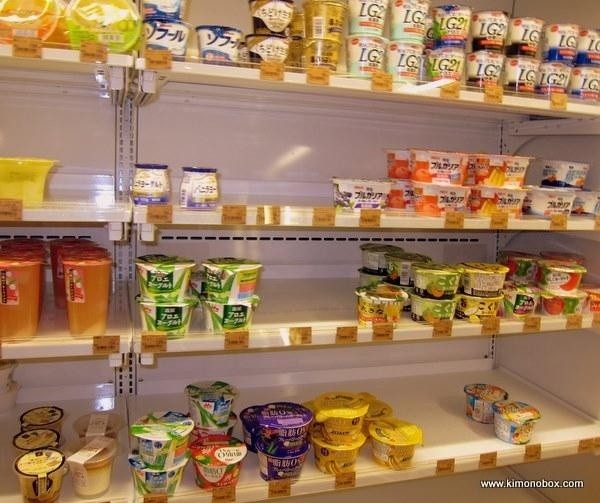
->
<box><xmin>0</xmin><ymin>47</ymin><xmax>600</xmax><ymax>503</ymax></box>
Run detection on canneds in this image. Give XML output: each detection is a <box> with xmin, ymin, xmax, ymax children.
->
<box><xmin>180</xmin><ymin>167</ymin><xmax>219</xmax><ymax>212</ymax></box>
<box><xmin>132</xmin><ymin>164</ymin><xmax>170</xmax><ymax>207</ymax></box>
